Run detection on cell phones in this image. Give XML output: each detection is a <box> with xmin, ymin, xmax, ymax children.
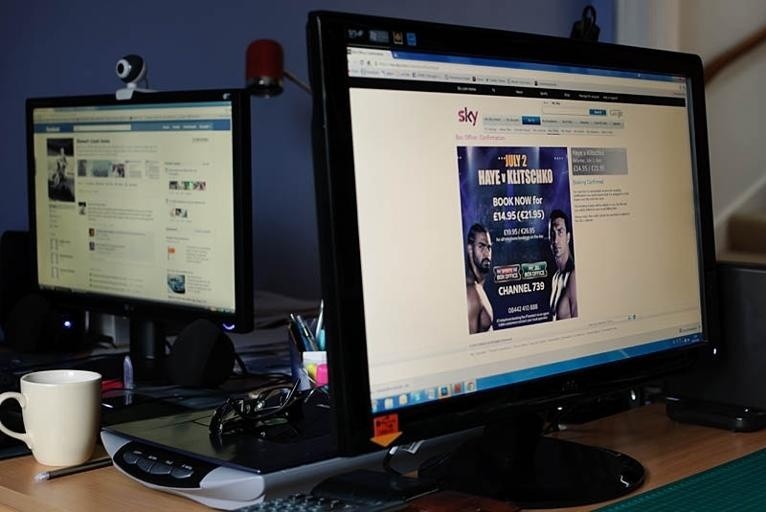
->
<box><xmin>665</xmin><ymin>398</ymin><xmax>766</xmax><ymax>434</ymax></box>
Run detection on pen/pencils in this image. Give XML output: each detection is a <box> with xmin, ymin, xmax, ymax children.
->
<box><xmin>288</xmin><ymin>299</ymin><xmax>327</xmax><ymax>351</ymax></box>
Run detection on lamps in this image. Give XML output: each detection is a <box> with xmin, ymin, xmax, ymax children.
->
<box><xmin>246</xmin><ymin>37</ymin><xmax>313</xmax><ymax>99</ymax></box>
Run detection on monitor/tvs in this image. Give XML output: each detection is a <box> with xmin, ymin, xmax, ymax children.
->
<box><xmin>25</xmin><ymin>89</ymin><xmax>270</xmax><ymax>413</ymax></box>
<box><xmin>304</xmin><ymin>9</ymin><xmax>730</xmax><ymax>509</ymax></box>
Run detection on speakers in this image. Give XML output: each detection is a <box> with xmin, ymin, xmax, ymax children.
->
<box><xmin>166</xmin><ymin>318</ymin><xmax>236</xmax><ymax>399</ymax></box>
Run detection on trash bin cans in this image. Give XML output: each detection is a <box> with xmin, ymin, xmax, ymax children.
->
<box><xmin>34</xmin><ymin>458</ymin><xmax>113</xmax><ymax>481</ymax></box>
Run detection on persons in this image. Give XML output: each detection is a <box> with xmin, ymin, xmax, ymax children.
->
<box><xmin>465</xmin><ymin>221</ymin><xmax>497</xmax><ymax>334</ymax></box>
<box><xmin>544</xmin><ymin>204</ymin><xmax>580</xmax><ymax>321</ymax></box>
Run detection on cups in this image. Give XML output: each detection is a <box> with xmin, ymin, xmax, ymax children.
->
<box><xmin>0</xmin><ymin>368</ymin><xmax>102</xmax><ymax>468</ymax></box>
